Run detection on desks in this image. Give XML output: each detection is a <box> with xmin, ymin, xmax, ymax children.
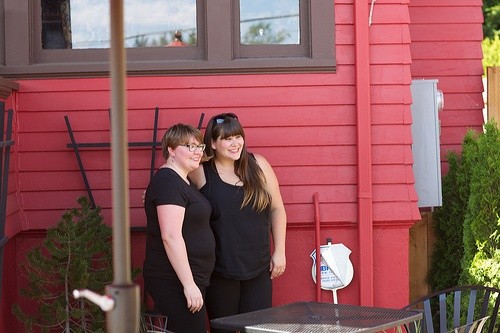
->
<box><xmin>210</xmin><ymin>301</ymin><xmax>423</xmax><ymax>333</ymax></box>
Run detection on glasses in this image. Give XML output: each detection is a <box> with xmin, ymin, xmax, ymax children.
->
<box><xmin>211</xmin><ymin>113</ymin><xmax>238</xmax><ymax>131</ymax></box>
<box><xmin>178</xmin><ymin>144</ymin><xmax>206</xmax><ymax>152</ymax></box>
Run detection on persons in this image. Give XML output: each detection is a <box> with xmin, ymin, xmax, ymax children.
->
<box><xmin>143</xmin><ymin>112</ymin><xmax>287</xmax><ymax>333</ymax></box>
<box><xmin>145</xmin><ymin>122</ymin><xmax>216</xmax><ymax>333</ymax></box>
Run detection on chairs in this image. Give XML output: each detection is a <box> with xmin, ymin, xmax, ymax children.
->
<box><xmin>400</xmin><ymin>285</ymin><xmax>500</xmax><ymax>333</ymax></box>
